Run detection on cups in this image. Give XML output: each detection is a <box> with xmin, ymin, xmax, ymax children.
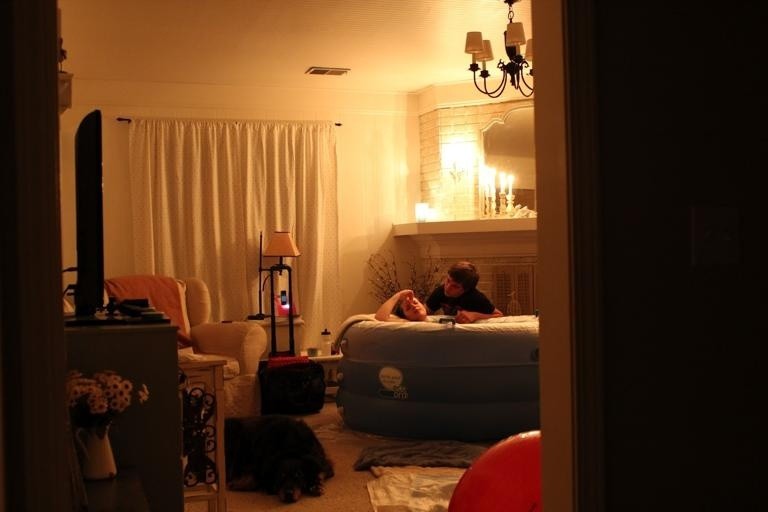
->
<box><xmin>307</xmin><ymin>348</ymin><xmax>318</xmax><ymax>357</ymax></box>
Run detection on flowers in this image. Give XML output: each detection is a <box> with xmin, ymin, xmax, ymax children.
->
<box><xmin>64</xmin><ymin>369</ymin><xmax>149</xmax><ymax>427</ymax></box>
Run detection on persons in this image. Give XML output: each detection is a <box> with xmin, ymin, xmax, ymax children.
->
<box><xmin>424</xmin><ymin>260</ymin><xmax>503</xmax><ymax>324</ymax></box>
<box><xmin>375</xmin><ymin>289</ymin><xmax>429</xmax><ymax>323</ymax></box>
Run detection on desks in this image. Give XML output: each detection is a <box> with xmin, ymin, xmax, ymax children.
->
<box><xmin>177</xmin><ymin>360</ymin><xmax>228</xmax><ymax>512</ymax></box>
<box><xmin>81</xmin><ymin>468</ymin><xmax>150</xmax><ymax>512</ymax></box>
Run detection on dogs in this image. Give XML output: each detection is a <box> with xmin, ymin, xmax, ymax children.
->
<box><xmin>223</xmin><ymin>414</ymin><xmax>335</xmax><ymax>505</ymax></box>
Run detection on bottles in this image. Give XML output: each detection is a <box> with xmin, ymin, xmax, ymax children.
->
<box><xmin>320</xmin><ymin>328</ymin><xmax>331</xmax><ymax>356</ymax></box>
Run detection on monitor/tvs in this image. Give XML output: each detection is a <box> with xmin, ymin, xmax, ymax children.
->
<box><xmin>71</xmin><ymin>109</ymin><xmax>105</xmax><ymax>318</ymax></box>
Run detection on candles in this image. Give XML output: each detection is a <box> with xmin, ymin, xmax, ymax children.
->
<box><xmin>485</xmin><ymin>166</ymin><xmax>515</xmax><ymax>201</ymax></box>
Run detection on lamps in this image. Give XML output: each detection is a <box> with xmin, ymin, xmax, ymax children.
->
<box><xmin>465</xmin><ymin>0</ymin><xmax>534</xmax><ymax>98</ymax></box>
<box><xmin>248</xmin><ymin>230</ymin><xmax>301</xmax><ymax>320</ymax></box>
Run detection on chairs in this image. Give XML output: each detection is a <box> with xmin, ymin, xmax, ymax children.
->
<box><xmin>103</xmin><ymin>276</ymin><xmax>268</xmax><ymax>419</ymax></box>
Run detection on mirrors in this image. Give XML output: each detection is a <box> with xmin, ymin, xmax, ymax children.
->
<box><xmin>479</xmin><ymin>105</ymin><xmax>536</xmax><ymax>217</ymax></box>
